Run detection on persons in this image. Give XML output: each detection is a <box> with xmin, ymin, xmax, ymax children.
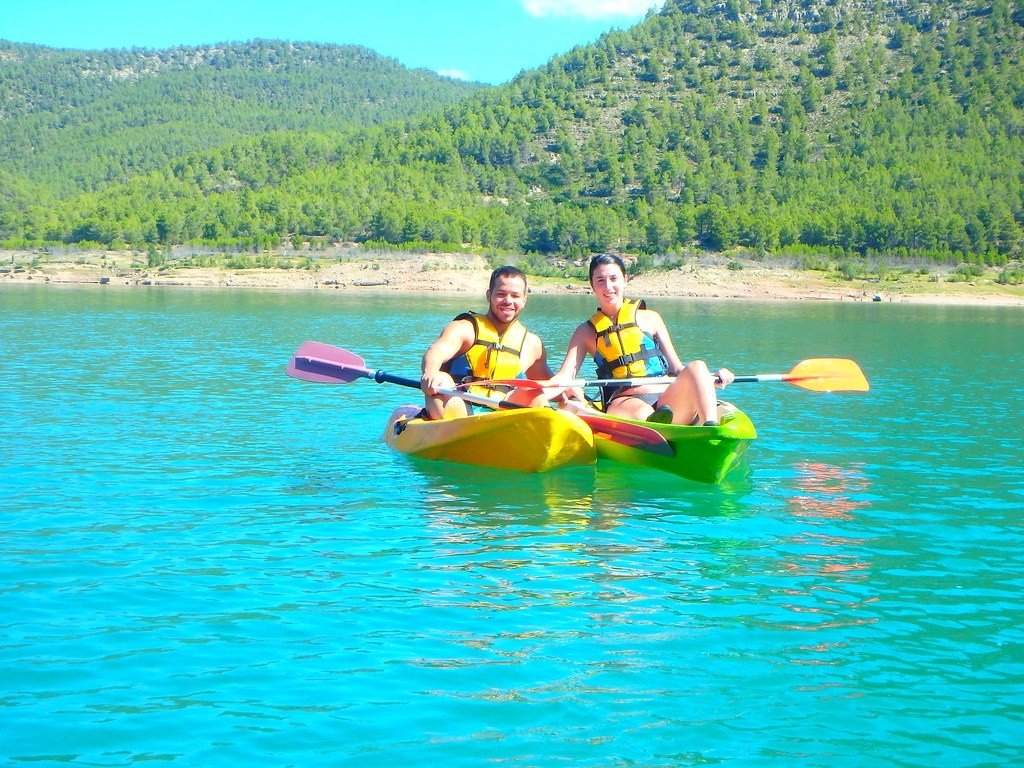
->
<box><xmin>422</xmin><ymin>265</ymin><xmax>584</xmax><ymax>421</ymax></box>
<box><xmin>502</xmin><ymin>254</ymin><xmax>735</xmax><ymax>426</ymax></box>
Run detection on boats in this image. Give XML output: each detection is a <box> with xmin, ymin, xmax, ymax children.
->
<box><xmin>872</xmin><ymin>295</ymin><xmax>881</xmax><ymax>301</ymax></box>
<box><xmin>566</xmin><ymin>394</ymin><xmax>757</xmax><ymax>489</ymax></box>
<box><xmin>380</xmin><ymin>405</ymin><xmax>598</xmax><ymax>469</ymax></box>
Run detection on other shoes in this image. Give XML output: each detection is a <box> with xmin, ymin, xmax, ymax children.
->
<box><xmin>647</xmin><ymin>404</ymin><xmax>674</xmax><ymax>424</ymax></box>
<box><xmin>703</xmin><ymin>421</ymin><xmax>718</xmax><ymax>426</ymax></box>
<box><xmin>445</xmin><ymin>396</ymin><xmax>469</xmax><ymax>423</ymax></box>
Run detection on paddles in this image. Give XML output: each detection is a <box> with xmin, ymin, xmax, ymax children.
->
<box><xmin>459</xmin><ymin>356</ymin><xmax>871</xmax><ymax>392</ymax></box>
<box><xmin>286</xmin><ymin>338</ymin><xmax>677</xmax><ymax>457</ymax></box>
